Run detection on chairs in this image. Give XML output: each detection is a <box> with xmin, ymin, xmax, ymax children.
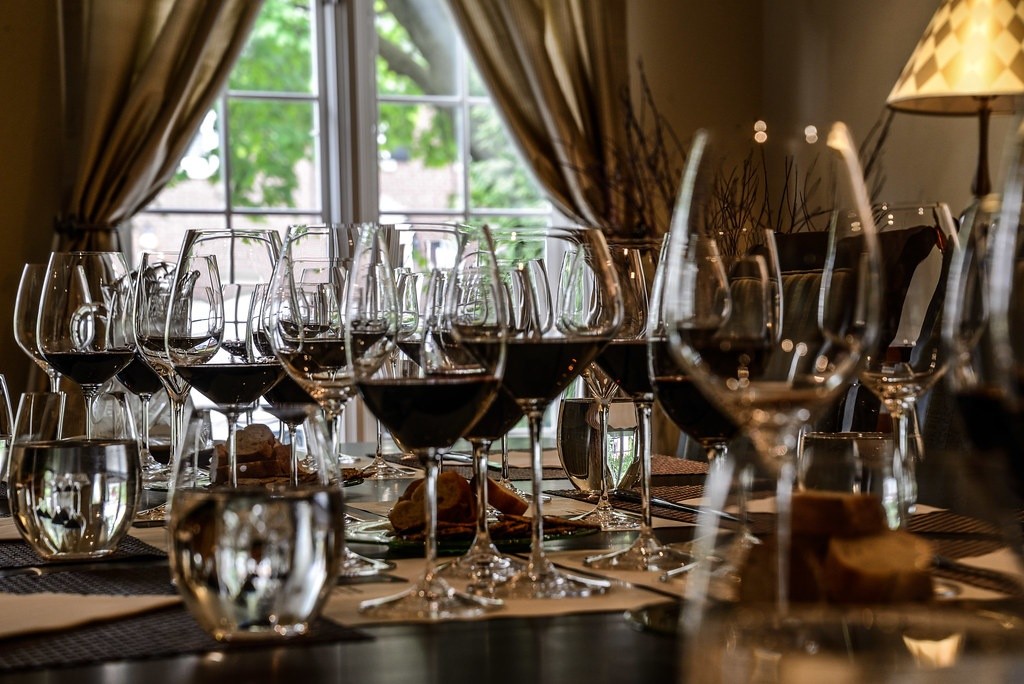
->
<box><xmin>777</xmin><ymin>225</ymin><xmax>955</xmax><ymax>503</ymax></box>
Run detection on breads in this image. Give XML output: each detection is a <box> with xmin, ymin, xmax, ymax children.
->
<box><xmin>207</xmin><ymin>424</ymin><xmax>309</xmax><ymax>482</ymax></box>
<box><xmin>738</xmin><ymin>488</ymin><xmax>934</xmax><ymax>606</ymax></box>
<box><xmin>385</xmin><ymin>469</ymin><xmax>529</xmax><ymax>536</ymax></box>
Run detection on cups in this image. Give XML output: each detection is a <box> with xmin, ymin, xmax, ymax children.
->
<box><xmin>0</xmin><ymin>374</ymin><xmax>14</xmax><ymax>484</ymax></box>
<box><xmin>557</xmin><ymin>398</ymin><xmax>661</xmax><ymax>497</ymax></box>
<box><xmin>166</xmin><ymin>410</ymin><xmax>345</xmax><ymax>642</ymax></box>
<box><xmin>7</xmin><ymin>393</ymin><xmax>144</xmax><ymax>561</ymax></box>
<box><xmin>87</xmin><ymin>378</ymin><xmax>195</xmax><ymax>515</ymax></box>
<box><xmin>800</xmin><ymin>431</ymin><xmax>908</xmax><ymax>532</ymax></box>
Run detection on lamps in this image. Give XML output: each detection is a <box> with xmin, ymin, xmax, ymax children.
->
<box><xmin>887</xmin><ymin>0</ymin><xmax>1024</xmax><ymax>199</ymax></box>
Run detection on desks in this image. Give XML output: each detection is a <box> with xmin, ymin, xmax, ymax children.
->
<box><xmin>0</xmin><ymin>447</ymin><xmax>1024</xmax><ymax>684</ymax></box>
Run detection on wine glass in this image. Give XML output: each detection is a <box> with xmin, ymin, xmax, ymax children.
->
<box><xmin>0</xmin><ymin>123</ymin><xmax>1024</xmax><ymax>684</ymax></box>
